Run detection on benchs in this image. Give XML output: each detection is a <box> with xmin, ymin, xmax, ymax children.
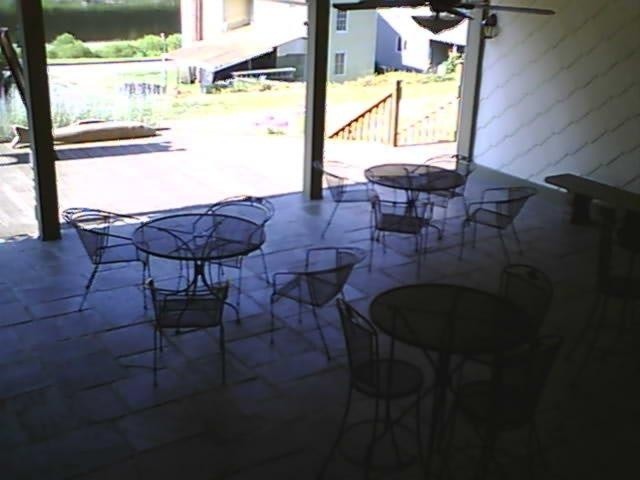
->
<box><xmin>544</xmin><ymin>172</ymin><xmax>639</xmax><ymax>243</ymax></box>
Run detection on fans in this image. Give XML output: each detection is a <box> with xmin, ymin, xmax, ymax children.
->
<box><xmin>332</xmin><ymin>1</ymin><xmax>555</xmax><ymax>18</ymax></box>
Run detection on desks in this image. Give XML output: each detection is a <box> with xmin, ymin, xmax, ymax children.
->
<box><xmin>364</xmin><ymin>163</ymin><xmax>463</xmax><ymax>243</ymax></box>
<box><xmin>131</xmin><ymin>213</ymin><xmax>267</xmax><ymax>334</ymax></box>
<box><xmin>369</xmin><ymin>283</ymin><xmax>527</xmax><ymax>476</ymax></box>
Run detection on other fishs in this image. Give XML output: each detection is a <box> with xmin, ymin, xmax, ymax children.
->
<box><xmin>11</xmin><ymin>120</ymin><xmax>157</xmax><ymax>149</ymax></box>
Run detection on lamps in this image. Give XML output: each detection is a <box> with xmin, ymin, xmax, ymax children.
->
<box><xmin>412</xmin><ymin>2</ymin><xmax>465</xmax><ymax>34</ymax></box>
<box><xmin>479</xmin><ymin>13</ymin><xmax>500</xmax><ymax>39</ymax></box>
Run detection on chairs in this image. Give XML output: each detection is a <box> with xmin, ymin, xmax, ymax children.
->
<box><xmin>459</xmin><ymin>185</ymin><xmax>539</xmax><ymax>272</ymax></box>
<box><xmin>269</xmin><ymin>246</ymin><xmax>369</xmax><ymax>360</ymax></box>
<box><xmin>321</xmin><ymin>298</ymin><xmax>427</xmax><ymax>477</ymax></box>
<box><xmin>142</xmin><ymin>278</ymin><xmax>230</xmax><ymax>391</ymax></box>
<box><xmin>61</xmin><ymin>208</ymin><xmax>152</xmax><ymax>312</ymax></box>
<box><xmin>194</xmin><ymin>195</ymin><xmax>274</xmax><ymax>321</ymax></box>
<box><xmin>315</xmin><ymin>160</ymin><xmax>382</xmax><ymax>242</ymax></box>
<box><xmin>369</xmin><ymin>195</ymin><xmax>433</xmax><ymax>279</ymax></box>
<box><xmin>571</xmin><ymin>224</ymin><xmax>638</xmax><ymax>379</ymax></box>
<box><xmin>410</xmin><ymin>156</ymin><xmax>478</xmax><ymax>240</ymax></box>
<box><xmin>468</xmin><ymin>263</ymin><xmax>554</xmax><ymax>389</ymax></box>
<box><xmin>433</xmin><ymin>334</ymin><xmax>564</xmax><ymax>476</ymax></box>
